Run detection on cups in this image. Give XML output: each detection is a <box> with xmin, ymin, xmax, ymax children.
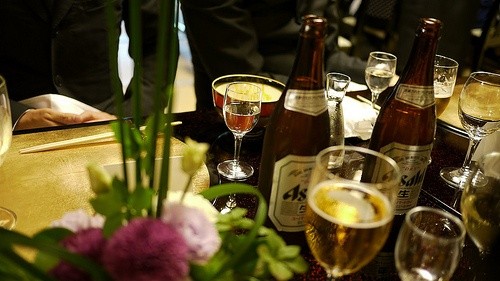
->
<box><xmin>393</xmin><ymin>206</ymin><xmax>466</xmax><ymax>281</ymax></box>
<box><xmin>304</xmin><ymin>146</ymin><xmax>402</xmax><ymax>281</ymax></box>
<box><xmin>431</xmin><ymin>53</ymin><xmax>458</xmax><ymax>121</ymax></box>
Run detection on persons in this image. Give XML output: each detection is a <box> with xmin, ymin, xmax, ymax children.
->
<box><xmin>182</xmin><ymin>0</ymin><xmax>358</xmax><ymax>79</ymax></box>
<box><xmin>0</xmin><ymin>0</ymin><xmax>179</xmax><ymax>129</ymax></box>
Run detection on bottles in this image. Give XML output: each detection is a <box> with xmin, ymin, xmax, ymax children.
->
<box><xmin>325</xmin><ymin>73</ymin><xmax>351</xmax><ymax>169</ymax></box>
<box><xmin>262</xmin><ymin>15</ymin><xmax>331</xmax><ymax>260</ymax></box>
<box><xmin>357</xmin><ymin>17</ymin><xmax>437</xmax><ymax>258</ymax></box>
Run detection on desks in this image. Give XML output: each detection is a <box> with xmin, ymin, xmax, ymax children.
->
<box><xmin>0</xmin><ymin>74</ymin><xmax>500</xmax><ymax>281</ymax></box>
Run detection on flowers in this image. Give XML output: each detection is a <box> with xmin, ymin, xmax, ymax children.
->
<box><xmin>0</xmin><ymin>0</ymin><xmax>309</xmax><ymax>281</ymax></box>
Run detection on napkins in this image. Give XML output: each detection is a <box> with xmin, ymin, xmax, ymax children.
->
<box><xmin>18</xmin><ymin>93</ymin><xmax>100</xmax><ymax>115</ymax></box>
<box><xmin>325</xmin><ymin>91</ymin><xmax>381</xmax><ymax>139</ymax></box>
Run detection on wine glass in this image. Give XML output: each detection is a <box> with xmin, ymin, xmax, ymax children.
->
<box><xmin>213</xmin><ymin>76</ymin><xmax>286</xmax><ymax>152</ymax></box>
<box><xmin>439</xmin><ymin>72</ymin><xmax>500</xmax><ymax>190</ymax></box>
<box><xmin>366</xmin><ymin>52</ymin><xmax>396</xmax><ymax>108</ymax></box>
<box><xmin>0</xmin><ymin>76</ymin><xmax>17</xmax><ymax>231</ymax></box>
<box><xmin>463</xmin><ymin>129</ymin><xmax>500</xmax><ymax>281</ymax></box>
<box><xmin>217</xmin><ymin>82</ymin><xmax>261</xmax><ymax>181</ymax></box>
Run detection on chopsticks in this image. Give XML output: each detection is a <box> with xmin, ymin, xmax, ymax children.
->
<box><xmin>18</xmin><ymin>120</ymin><xmax>183</xmax><ymax>154</ymax></box>
<box><xmin>344</xmin><ymin>95</ymin><xmax>382</xmax><ymax>114</ymax></box>
